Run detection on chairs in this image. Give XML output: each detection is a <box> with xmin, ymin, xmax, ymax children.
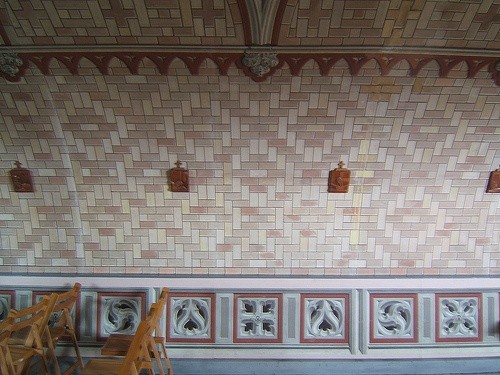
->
<box><xmin>0</xmin><ymin>281</ymin><xmax>173</xmax><ymax>375</ymax></box>
